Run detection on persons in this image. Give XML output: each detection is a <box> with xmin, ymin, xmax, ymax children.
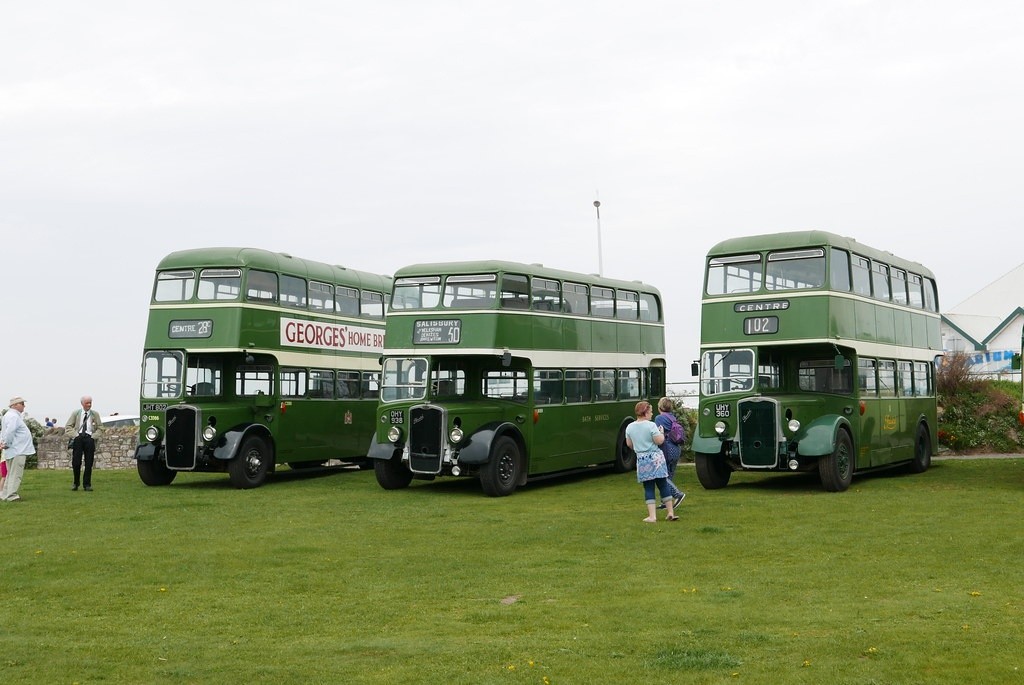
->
<box><xmin>625</xmin><ymin>401</ymin><xmax>680</xmax><ymax>523</ymax></box>
<box><xmin>65</xmin><ymin>396</ymin><xmax>105</xmax><ymax>491</ymax></box>
<box><xmin>45</xmin><ymin>417</ymin><xmax>59</xmax><ymax>428</ymax></box>
<box><xmin>0</xmin><ymin>397</ymin><xmax>36</xmax><ymax>501</ymax></box>
<box><xmin>656</xmin><ymin>397</ymin><xmax>686</xmax><ymax>508</ymax></box>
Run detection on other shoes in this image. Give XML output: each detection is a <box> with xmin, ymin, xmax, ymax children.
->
<box><xmin>6</xmin><ymin>492</ymin><xmax>20</xmax><ymax>501</ymax></box>
<box><xmin>72</xmin><ymin>486</ymin><xmax>78</xmax><ymax>491</ymax></box>
<box><xmin>656</xmin><ymin>504</ymin><xmax>667</xmax><ymax>510</ymax></box>
<box><xmin>84</xmin><ymin>487</ymin><xmax>93</xmax><ymax>491</ymax></box>
<box><xmin>666</xmin><ymin>512</ymin><xmax>673</xmax><ymax>521</ymax></box>
<box><xmin>673</xmin><ymin>492</ymin><xmax>685</xmax><ymax>508</ymax></box>
<box><xmin>643</xmin><ymin>516</ymin><xmax>656</xmax><ymax>522</ymax></box>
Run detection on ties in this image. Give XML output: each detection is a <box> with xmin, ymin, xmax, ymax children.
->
<box><xmin>82</xmin><ymin>412</ymin><xmax>88</xmax><ymax>437</ymax></box>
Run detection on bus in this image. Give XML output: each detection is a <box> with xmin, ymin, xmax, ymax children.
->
<box><xmin>691</xmin><ymin>229</ymin><xmax>944</xmax><ymax>492</ymax></box>
<box><xmin>133</xmin><ymin>246</ymin><xmax>422</xmax><ymax>489</ymax></box>
<box><xmin>366</xmin><ymin>260</ymin><xmax>667</xmax><ymax>496</ymax></box>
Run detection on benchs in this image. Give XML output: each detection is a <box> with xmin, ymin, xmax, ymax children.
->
<box><xmin>451</xmin><ymin>298</ymin><xmax>649</xmax><ymax>323</ymax></box>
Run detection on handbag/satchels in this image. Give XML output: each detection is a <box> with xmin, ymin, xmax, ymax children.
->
<box><xmin>68</xmin><ymin>438</ymin><xmax>74</xmax><ymax>450</ymax></box>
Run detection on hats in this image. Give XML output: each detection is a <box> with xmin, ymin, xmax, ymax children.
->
<box><xmin>8</xmin><ymin>395</ymin><xmax>27</xmax><ymax>406</ymax></box>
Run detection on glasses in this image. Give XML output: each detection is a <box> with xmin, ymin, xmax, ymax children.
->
<box><xmin>21</xmin><ymin>403</ymin><xmax>25</xmax><ymax>405</ymax></box>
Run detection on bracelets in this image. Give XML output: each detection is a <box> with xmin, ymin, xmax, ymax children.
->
<box><xmin>660</xmin><ymin>432</ymin><xmax>664</xmax><ymax>434</ymax></box>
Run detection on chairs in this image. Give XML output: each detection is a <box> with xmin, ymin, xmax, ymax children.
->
<box><xmin>746</xmin><ymin>376</ymin><xmax>771</xmax><ymax>390</ymax></box>
<box><xmin>433</xmin><ymin>381</ymin><xmax>455</xmax><ymax>397</ymax></box>
<box><xmin>191</xmin><ymin>382</ymin><xmax>215</xmax><ymax>396</ymax></box>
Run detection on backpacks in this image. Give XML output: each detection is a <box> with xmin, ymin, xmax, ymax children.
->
<box><xmin>662</xmin><ymin>413</ymin><xmax>686</xmax><ymax>445</ymax></box>
